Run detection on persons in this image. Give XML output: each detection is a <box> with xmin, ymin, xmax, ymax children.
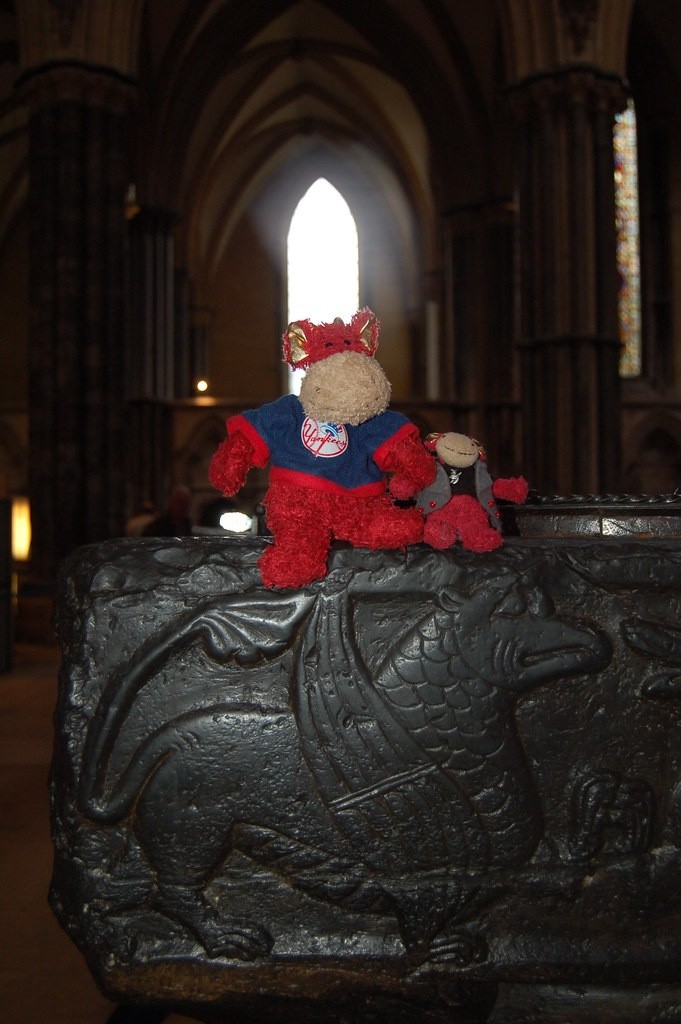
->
<box><xmin>124</xmin><ymin>486</ymin><xmax>192</xmax><ymax>538</ymax></box>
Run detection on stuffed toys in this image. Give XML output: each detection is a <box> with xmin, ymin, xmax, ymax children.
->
<box><xmin>414</xmin><ymin>432</ymin><xmax>528</xmax><ymax>551</ymax></box>
<box><xmin>208</xmin><ymin>308</ymin><xmax>438</xmax><ymax>589</ymax></box>
